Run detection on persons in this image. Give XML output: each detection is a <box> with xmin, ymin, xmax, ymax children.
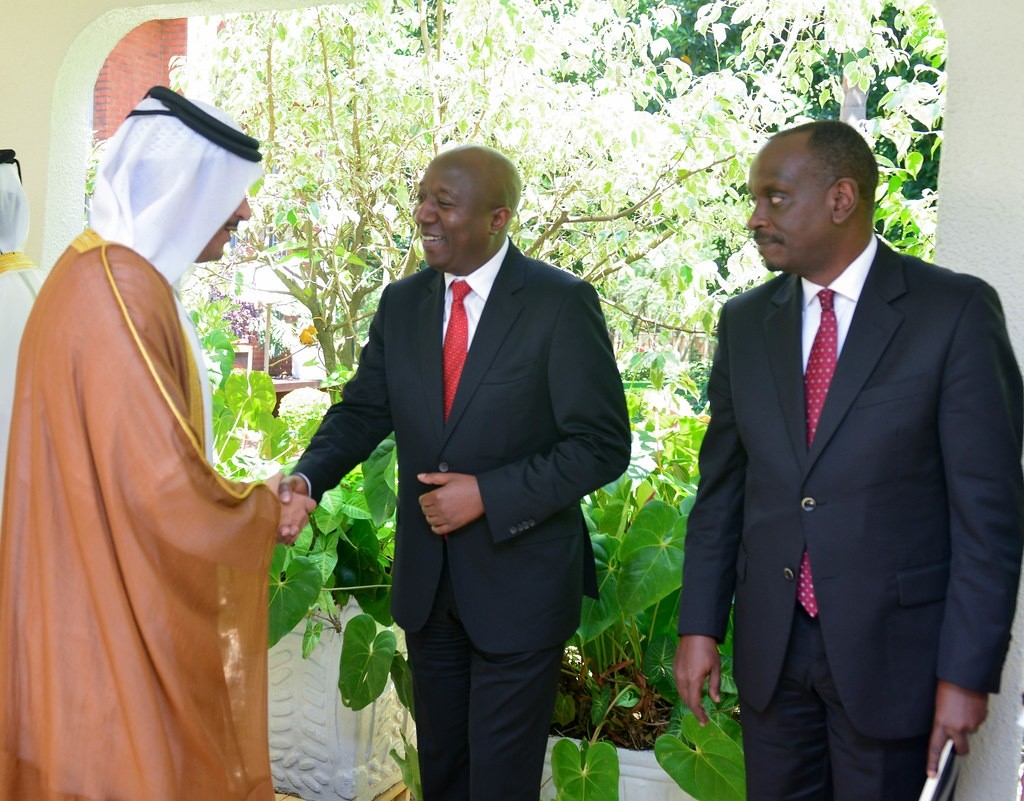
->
<box><xmin>3</xmin><ymin>87</ymin><xmax>316</xmax><ymax>800</ymax></box>
<box><xmin>1</xmin><ymin>145</ymin><xmax>54</xmax><ymax>480</ymax></box>
<box><xmin>275</xmin><ymin>148</ymin><xmax>631</xmax><ymax>800</ymax></box>
<box><xmin>676</xmin><ymin>121</ymin><xmax>1023</xmax><ymax>799</ymax></box>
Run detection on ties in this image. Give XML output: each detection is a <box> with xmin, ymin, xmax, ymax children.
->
<box><xmin>797</xmin><ymin>289</ymin><xmax>836</xmax><ymax>618</ymax></box>
<box><xmin>445</xmin><ymin>281</ymin><xmax>471</xmax><ymax>421</ymax></box>
